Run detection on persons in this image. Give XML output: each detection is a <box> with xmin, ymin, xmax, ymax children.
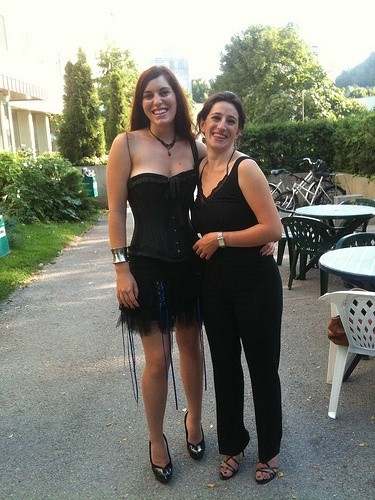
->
<box><xmin>194</xmin><ymin>91</ymin><xmax>283</xmax><ymax>484</ymax></box>
<box><xmin>106</xmin><ymin>66</ymin><xmax>275</xmax><ymax>483</ymax></box>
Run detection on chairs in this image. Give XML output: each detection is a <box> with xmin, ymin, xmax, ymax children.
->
<box><xmin>274</xmin><ymin>198</ymin><xmax>375</xmax><ymax>417</ymax></box>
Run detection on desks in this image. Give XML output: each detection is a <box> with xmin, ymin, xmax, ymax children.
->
<box><xmin>318</xmin><ymin>246</ymin><xmax>375</xmax><ymax>382</ymax></box>
<box><xmin>294</xmin><ymin>204</ymin><xmax>375</xmax><ymax>281</ymax></box>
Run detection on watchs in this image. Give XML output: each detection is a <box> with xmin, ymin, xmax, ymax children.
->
<box><xmin>217</xmin><ymin>231</ymin><xmax>225</xmax><ymax>247</ymax></box>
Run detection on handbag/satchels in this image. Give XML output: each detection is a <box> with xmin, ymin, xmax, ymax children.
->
<box><xmin>327</xmin><ymin>287</ymin><xmax>373</xmax><ymax>346</ymax></box>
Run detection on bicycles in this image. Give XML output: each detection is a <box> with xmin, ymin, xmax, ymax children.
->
<box><xmin>269</xmin><ymin>157</ymin><xmax>349</xmax><ymax>217</ymax></box>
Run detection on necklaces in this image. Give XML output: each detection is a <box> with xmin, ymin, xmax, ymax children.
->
<box><xmin>148</xmin><ymin>127</ymin><xmax>177</xmax><ymax>156</ymax></box>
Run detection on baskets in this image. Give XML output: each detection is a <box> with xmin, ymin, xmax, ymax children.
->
<box><xmin>311</xmin><ymin>164</ymin><xmax>330</xmax><ymax>178</ymax></box>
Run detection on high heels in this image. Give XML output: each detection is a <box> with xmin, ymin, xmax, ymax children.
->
<box><xmin>219</xmin><ymin>449</ymin><xmax>244</xmax><ymax>480</ymax></box>
<box><xmin>183</xmin><ymin>412</ymin><xmax>205</xmax><ymax>460</ymax></box>
<box><xmin>256</xmin><ymin>453</ymin><xmax>280</xmax><ymax>484</ymax></box>
<box><xmin>148</xmin><ymin>433</ymin><xmax>173</xmax><ymax>483</ymax></box>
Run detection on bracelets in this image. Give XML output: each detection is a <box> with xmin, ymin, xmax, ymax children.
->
<box><xmin>111</xmin><ymin>247</ymin><xmax>128</xmax><ymax>264</ymax></box>
